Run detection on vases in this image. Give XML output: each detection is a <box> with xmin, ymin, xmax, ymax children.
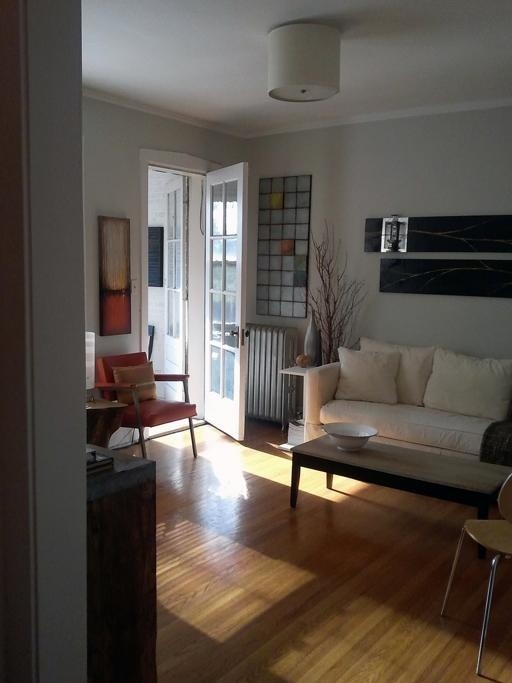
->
<box><xmin>304</xmin><ymin>310</ymin><xmax>322</xmax><ymax>367</ymax></box>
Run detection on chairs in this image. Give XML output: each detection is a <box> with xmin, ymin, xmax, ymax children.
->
<box><xmin>440</xmin><ymin>474</ymin><xmax>512</xmax><ymax>675</ymax></box>
<box><xmin>94</xmin><ymin>351</ymin><xmax>198</xmax><ymax>458</ymax></box>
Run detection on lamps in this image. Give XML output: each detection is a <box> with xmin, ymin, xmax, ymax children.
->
<box><xmin>85</xmin><ymin>332</ymin><xmax>96</xmax><ymax>402</ymax></box>
<box><xmin>266</xmin><ymin>23</ymin><xmax>342</xmax><ymax>101</ymax></box>
<box><xmin>387</xmin><ymin>215</ymin><xmax>401</xmax><ymax>251</ymax></box>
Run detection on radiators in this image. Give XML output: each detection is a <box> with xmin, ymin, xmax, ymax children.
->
<box><xmin>245</xmin><ymin>324</ymin><xmax>298</xmax><ymax>433</ymax></box>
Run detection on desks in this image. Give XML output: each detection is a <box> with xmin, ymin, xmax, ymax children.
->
<box><xmin>279</xmin><ymin>365</ymin><xmax>316</xmax><ymax>453</ymax></box>
<box><xmin>291</xmin><ymin>434</ymin><xmax>512</xmax><ymax>560</ymax></box>
<box><xmin>86</xmin><ymin>439</ymin><xmax>157</xmax><ymax>683</ymax></box>
<box><xmin>87</xmin><ymin>397</ymin><xmax>128</xmax><ymax>449</ymax></box>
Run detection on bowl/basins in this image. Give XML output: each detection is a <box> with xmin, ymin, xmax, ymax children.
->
<box><xmin>323</xmin><ymin>422</ymin><xmax>378</xmax><ymax>452</ymax></box>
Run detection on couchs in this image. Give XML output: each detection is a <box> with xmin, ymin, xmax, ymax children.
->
<box><xmin>304</xmin><ymin>361</ymin><xmax>512</xmax><ymax>467</ymax></box>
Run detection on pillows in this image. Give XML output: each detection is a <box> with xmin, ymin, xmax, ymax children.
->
<box><xmin>359</xmin><ymin>336</ymin><xmax>437</xmax><ymax>407</ymax></box>
<box><xmin>334</xmin><ymin>346</ymin><xmax>401</xmax><ymax>405</ymax></box>
<box><xmin>111</xmin><ymin>361</ymin><xmax>157</xmax><ymax>404</ymax></box>
<box><xmin>422</xmin><ymin>348</ymin><xmax>512</xmax><ymax>422</ymax></box>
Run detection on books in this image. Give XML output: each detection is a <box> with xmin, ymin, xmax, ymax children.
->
<box><xmin>86</xmin><ymin>449</ymin><xmax>114</xmax><ymax>476</ymax></box>
